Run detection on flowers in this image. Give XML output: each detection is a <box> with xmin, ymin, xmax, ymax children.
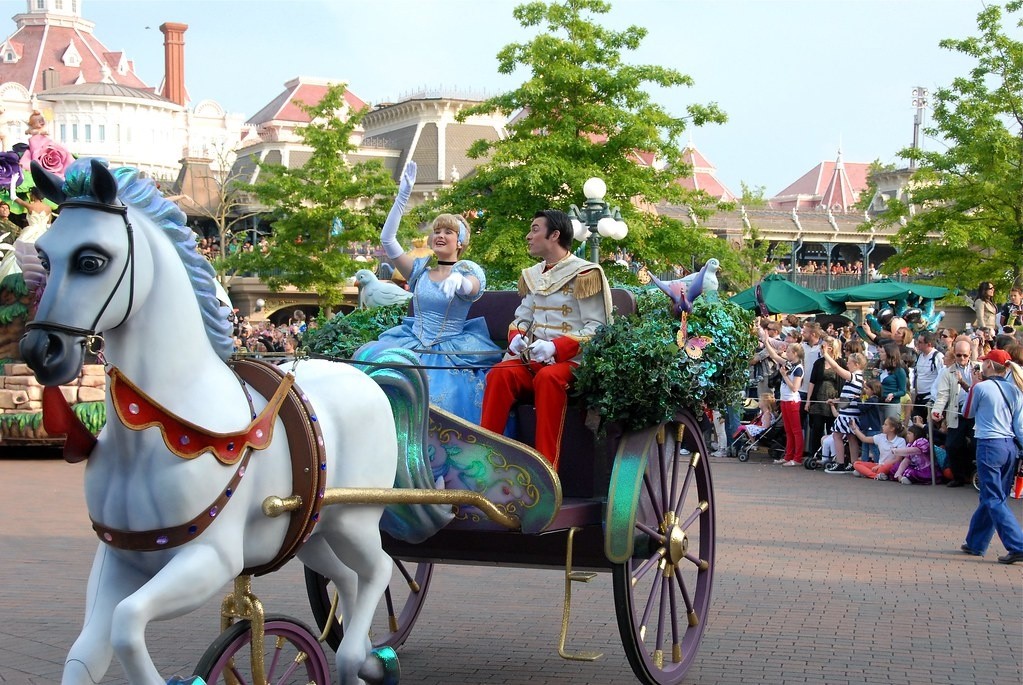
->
<box><xmin>0</xmin><ymin>134</ymin><xmax>76</xmax><ymax>188</ymax></box>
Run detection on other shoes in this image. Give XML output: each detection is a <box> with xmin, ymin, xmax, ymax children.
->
<box><xmin>773</xmin><ymin>458</ymin><xmax>787</xmax><ymax>465</ymax></box>
<box><xmin>743</xmin><ymin>445</ymin><xmax>759</xmax><ymax>452</ymax></box>
<box><xmin>782</xmin><ymin>460</ymin><xmax>803</xmax><ymax>467</ymax></box>
<box><xmin>824</xmin><ymin>462</ymin><xmax>969</xmax><ymax>488</ymax></box>
<box><xmin>711</xmin><ymin>450</ymin><xmax>727</xmax><ymax>458</ymax></box>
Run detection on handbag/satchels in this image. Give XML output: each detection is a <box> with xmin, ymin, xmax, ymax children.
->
<box><xmin>768</xmin><ymin>369</ymin><xmax>782</xmax><ymax>389</ymax></box>
<box><xmin>839</xmin><ymin>398</ymin><xmax>850</xmax><ymax>410</ymax></box>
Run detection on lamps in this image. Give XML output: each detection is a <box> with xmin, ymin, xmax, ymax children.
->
<box><xmin>255</xmin><ymin>299</ymin><xmax>265</xmax><ymax>312</ymax></box>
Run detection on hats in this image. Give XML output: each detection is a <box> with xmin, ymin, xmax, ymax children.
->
<box><xmin>978</xmin><ymin>349</ymin><xmax>1012</xmax><ymax>367</ymax></box>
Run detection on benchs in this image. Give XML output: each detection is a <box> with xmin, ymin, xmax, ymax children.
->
<box><xmin>408</xmin><ymin>288</ymin><xmax>636</xmax><ymax>495</ymax></box>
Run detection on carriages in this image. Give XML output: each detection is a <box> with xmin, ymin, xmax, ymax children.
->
<box><xmin>13</xmin><ymin>163</ymin><xmax>718</xmax><ymax>685</ymax></box>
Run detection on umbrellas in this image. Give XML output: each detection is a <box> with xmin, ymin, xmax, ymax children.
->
<box><xmin>728</xmin><ymin>273</ymin><xmax>847</xmax><ymax>315</ymax></box>
<box><xmin>821</xmin><ymin>274</ymin><xmax>960</xmax><ymax>303</ymax></box>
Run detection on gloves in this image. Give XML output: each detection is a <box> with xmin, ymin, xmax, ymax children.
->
<box><xmin>509</xmin><ymin>333</ymin><xmax>529</xmax><ymax>355</ymax></box>
<box><xmin>379</xmin><ymin>161</ymin><xmax>417</xmax><ymax>259</ymax></box>
<box><xmin>529</xmin><ymin>339</ymin><xmax>556</xmax><ymax>362</ymax></box>
<box><xmin>439</xmin><ymin>272</ymin><xmax>473</xmax><ymax>299</ymax></box>
<box><xmin>10</xmin><ymin>172</ymin><xmax>20</xmax><ymax>201</ymax></box>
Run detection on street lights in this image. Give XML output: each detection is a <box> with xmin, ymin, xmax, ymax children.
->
<box><xmin>564</xmin><ymin>176</ymin><xmax>628</xmax><ymax>273</ymax></box>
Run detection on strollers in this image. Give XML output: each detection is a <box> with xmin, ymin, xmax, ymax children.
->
<box><xmin>803</xmin><ymin>428</ymin><xmax>851</xmax><ymax>473</ymax></box>
<box><xmin>726</xmin><ymin>403</ymin><xmax>787</xmax><ymax>462</ymax></box>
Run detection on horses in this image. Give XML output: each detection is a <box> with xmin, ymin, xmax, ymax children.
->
<box><xmin>20</xmin><ymin>155</ymin><xmax>456</xmax><ymax>685</ymax></box>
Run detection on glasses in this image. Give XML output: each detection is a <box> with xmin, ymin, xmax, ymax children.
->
<box><xmin>955</xmin><ymin>351</ymin><xmax>970</xmax><ymax>358</ymax></box>
<box><xmin>987</xmin><ymin>287</ymin><xmax>994</xmax><ymax>290</ymax></box>
<box><xmin>919</xmin><ymin>340</ymin><xmax>922</xmax><ymax>343</ymax></box>
<box><xmin>941</xmin><ymin>334</ymin><xmax>948</xmax><ymax>338</ymax></box>
<box><xmin>767</xmin><ymin>326</ymin><xmax>850</xmax><ymax>352</ymax></box>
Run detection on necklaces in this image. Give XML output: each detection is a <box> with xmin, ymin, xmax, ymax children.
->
<box><xmin>438</xmin><ymin>261</ymin><xmax>456</xmax><ymax>265</ymax></box>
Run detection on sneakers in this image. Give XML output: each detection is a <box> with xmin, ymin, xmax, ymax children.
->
<box><xmin>961</xmin><ymin>544</ymin><xmax>984</xmax><ymax>557</ymax></box>
<box><xmin>998</xmin><ymin>552</ymin><xmax>1023</xmax><ymax>563</ymax></box>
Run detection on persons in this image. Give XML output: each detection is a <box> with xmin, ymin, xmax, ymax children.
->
<box><xmin>973</xmin><ymin>281</ymin><xmax>998</xmax><ymax>330</ymax></box>
<box><xmin>1000</xmin><ymin>287</ymin><xmax>1023</xmax><ymax>327</ymax></box>
<box><xmin>0</xmin><ymin>172</ymin><xmax>52</xmax><ymax>283</ymax></box>
<box><xmin>763</xmin><ymin>257</ymin><xmax>875</xmax><ymax>287</ymax></box>
<box><xmin>0</xmin><ymin>201</ymin><xmax>22</xmax><ymax>261</ymax></box>
<box><xmin>679</xmin><ymin>314</ymin><xmax>1023</xmax><ymax>484</ymax></box>
<box><xmin>345</xmin><ymin>160</ymin><xmax>516</xmax><ymax>436</ymax></box>
<box><xmin>961</xmin><ymin>350</ymin><xmax>1023</xmax><ymax>564</ymax></box>
<box><xmin>901</xmin><ymin>266</ymin><xmax>920</xmax><ymax>276</ymax></box>
<box><xmin>198</xmin><ymin>236</ymin><xmax>268</xmax><ymax>260</ymax></box>
<box><xmin>480</xmin><ymin>210</ymin><xmax>614</xmax><ymax>472</ymax></box>
<box><xmin>228</xmin><ymin>310</ymin><xmax>314</xmax><ymax>352</ymax></box>
